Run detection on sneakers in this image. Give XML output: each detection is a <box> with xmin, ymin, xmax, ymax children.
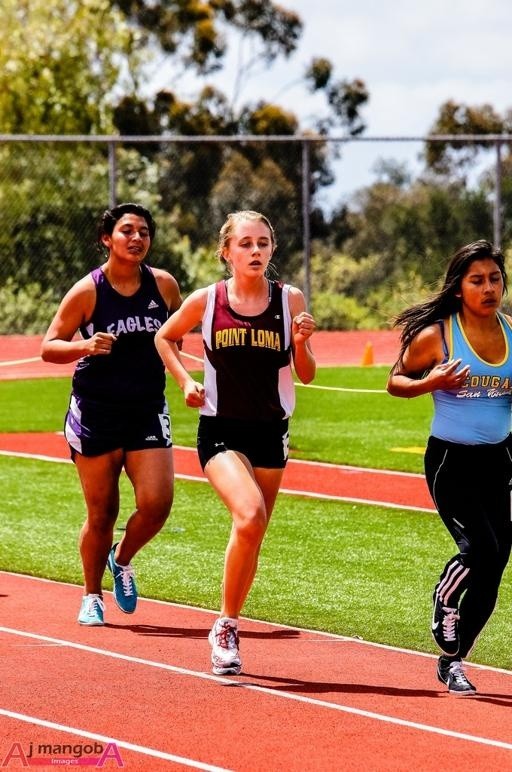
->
<box><xmin>431</xmin><ymin>589</ymin><xmax>461</xmax><ymax>656</ymax></box>
<box><xmin>437</xmin><ymin>656</ymin><xmax>476</xmax><ymax>696</ymax></box>
<box><xmin>207</xmin><ymin>616</ymin><xmax>242</xmax><ymax>675</ymax></box>
<box><xmin>106</xmin><ymin>542</ymin><xmax>139</xmax><ymax>614</ymax></box>
<box><xmin>77</xmin><ymin>594</ymin><xmax>105</xmax><ymax>626</ymax></box>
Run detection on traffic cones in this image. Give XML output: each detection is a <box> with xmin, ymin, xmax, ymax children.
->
<box><xmin>360</xmin><ymin>340</ymin><xmax>375</xmax><ymax>367</ymax></box>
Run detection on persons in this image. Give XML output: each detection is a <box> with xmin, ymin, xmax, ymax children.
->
<box><xmin>153</xmin><ymin>209</ymin><xmax>318</xmax><ymax>675</ymax></box>
<box><xmin>41</xmin><ymin>204</ymin><xmax>186</xmax><ymax>622</ymax></box>
<box><xmin>386</xmin><ymin>238</ymin><xmax>512</xmax><ymax>695</ymax></box>
